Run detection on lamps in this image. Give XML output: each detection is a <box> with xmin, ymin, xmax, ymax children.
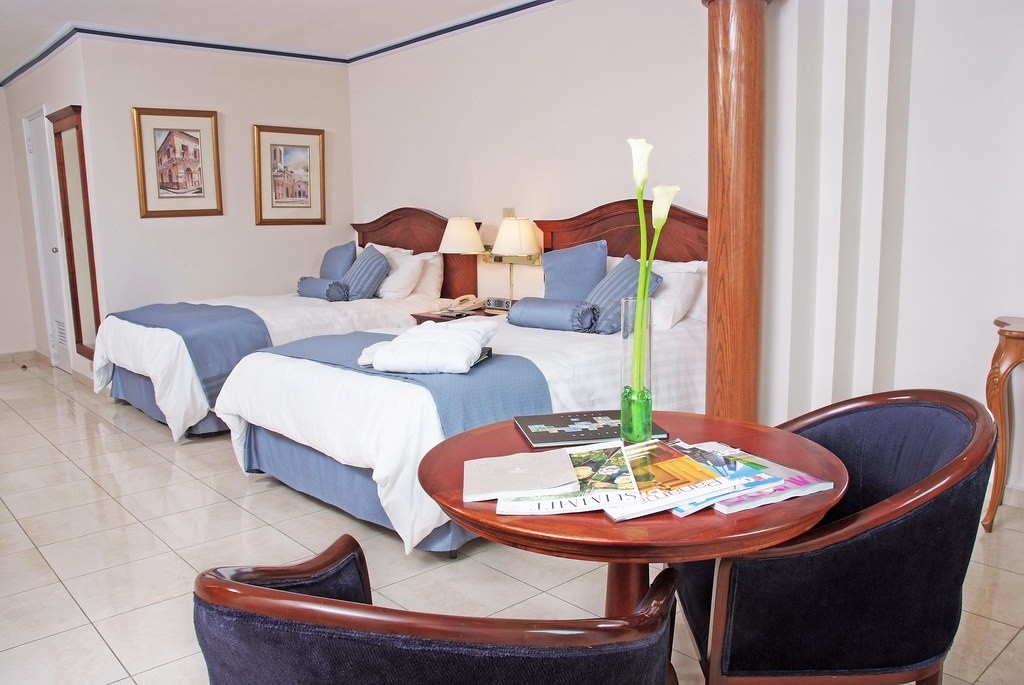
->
<box><xmin>438</xmin><ymin>216</ymin><xmax>502</xmax><ymax>262</ymax></box>
<box><xmin>492</xmin><ymin>215</ymin><xmax>542</xmax><ymax>266</ymax></box>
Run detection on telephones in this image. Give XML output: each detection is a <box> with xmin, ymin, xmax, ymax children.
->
<box><xmin>449</xmin><ymin>294</ymin><xmax>487</xmax><ymax>312</ymax></box>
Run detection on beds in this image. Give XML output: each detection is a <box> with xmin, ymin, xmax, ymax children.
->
<box><xmin>93</xmin><ymin>208</ymin><xmax>482</xmax><ymax>442</ymax></box>
<box><xmin>212</xmin><ymin>199</ymin><xmax>709</xmax><ymax>560</ymax></box>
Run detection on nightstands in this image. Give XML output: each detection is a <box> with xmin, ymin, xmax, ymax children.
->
<box><xmin>410</xmin><ymin>311</ymin><xmax>495</xmax><ymax>326</ymax></box>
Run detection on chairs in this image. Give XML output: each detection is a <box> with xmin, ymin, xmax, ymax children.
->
<box><xmin>667</xmin><ymin>388</ymin><xmax>998</xmax><ymax>685</ymax></box>
<box><xmin>192</xmin><ymin>534</ymin><xmax>683</xmax><ymax>685</ymax></box>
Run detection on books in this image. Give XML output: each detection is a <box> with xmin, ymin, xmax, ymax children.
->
<box><xmin>463</xmin><ymin>438</ymin><xmax>834</xmax><ymax>523</ymax></box>
<box><xmin>513</xmin><ymin>410</ymin><xmax>669</xmax><ymax>449</ymax></box>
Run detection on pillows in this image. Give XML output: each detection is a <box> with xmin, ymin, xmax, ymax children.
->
<box><xmin>296</xmin><ymin>240</ymin><xmax>444</xmax><ymax>302</ymax></box>
<box><xmin>507</xmin><ymin>240</ymin><xmax>709</xmax><ymax>335</ymax></box>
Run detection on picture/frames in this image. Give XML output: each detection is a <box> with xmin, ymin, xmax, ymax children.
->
<box><xmin>133</xmin><ymin>107</ymin><xmax>224</xmax><ymax>218</ymax></box>
<box><xmin>253</xmin><ymin>124</ymin><xmax>327</xmax><ymax>226</ymax></box>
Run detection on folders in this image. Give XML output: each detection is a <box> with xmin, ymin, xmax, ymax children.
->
<box><xmin>470</xmin><ymin>347</ymin><xmax>493</xmax><ymax>368</ymax></box>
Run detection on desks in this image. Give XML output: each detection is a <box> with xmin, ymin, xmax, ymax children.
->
<box><xmin>979</xmin><ymin>317</ymin><xmax>1024</xmax><ymax>532</ymax></box>
<box><xmin>419</xmin><ymin>412</ymin><xmax>849</xmax><ymax>685</ymax></box>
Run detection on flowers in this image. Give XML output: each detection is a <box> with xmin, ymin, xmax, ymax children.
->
<box><xmin>626</xmin><ymin>138</ymin><xmax>680</xmax><ymax>436</ymax></box>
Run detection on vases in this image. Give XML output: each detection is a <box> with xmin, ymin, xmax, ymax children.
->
<box><xmin>621</xmin><ymin>297</ymin><xmax>653</xmax><ymax>445</ymax></box>
